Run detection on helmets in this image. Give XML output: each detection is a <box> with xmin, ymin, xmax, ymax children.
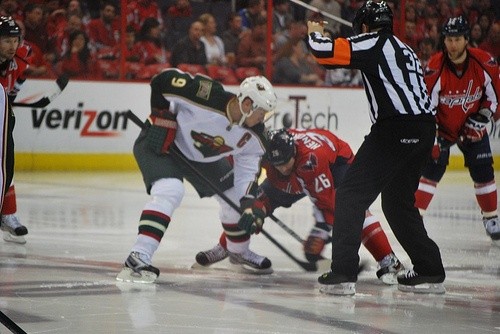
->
<box><xmin>267</xmin><ymin>128</ymin><xmax>294</xmax><ymax>166</ymax></box>
<box><xmin>442</xmin><ymin>15</ymin><xmax>469</xmax><ymax>40</ymax></box>
<box><xmin>237</xmin><ymin>76</ymin><xmax>277</xmax><ymax>117</ymax></box>
<box><xmin>0</xmin><ymin>16</ymin><xmax>22</xmax><ymax>37</ymax></box>
<box><xmin>352</xmin><ymin>0</ymin><xmax>394</xmax><ymax>35</ymax></box>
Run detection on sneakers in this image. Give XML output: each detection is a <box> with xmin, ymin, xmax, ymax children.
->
<box><xmin>318</xmin><ymin>272</ymin><xmax>357</xmax><ymax>295</ymax></box>
<box><xmin>228</xmin><ymin>249</ymin><xmax>273</xmax><ymax>274</ymax></box>
<box><xmin>116</xmin><ymin>251</ymin><xmax>160</xmax><ymax>283</ymax></box>
<box><xmin>396</xmin><ymin>269</ymin><xmax>446</xmax><ymax>295</ymax></box>
<box><xmin>191</xmin><ymin>241</ymin><xmax>228</xmax><ymax>268</ymax></box>
<box><xmin>482</xmin><ymin>216</ymin><xmax>500</xmax><ymax>248</ymax></box>
<box><xmin>376</xmin><ymin>251</ymin><xmax>405</xmax><ymax>285</ymax></box>
<box><xmin>0</xmin><ymin>214</ymin><xmax>28</xmax><ymax>244</ymax></box>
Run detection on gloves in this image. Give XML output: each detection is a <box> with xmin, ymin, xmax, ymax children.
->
<box><xmin>432</xmin><ymin>123</ymin><xmax>439</xmax><ymax>159</ymax></box>
<box><xmin>465</xmin><ymin>108</ymin><xmax>493</xmax><ymax>142</ymax></box>
<box><xmin>304</xmin><ymin>223</ymin><xmax>332</xmax><ymax>263</ymax></box>
<box><xmin>237</xmin><ymin>197</ymin><xmax>266</xmax><ymax>234</ymax></box>
<box><xmin>147</xmin><ymin>109</ymin><xmax>177</xmax><ymax>156</ymax></box>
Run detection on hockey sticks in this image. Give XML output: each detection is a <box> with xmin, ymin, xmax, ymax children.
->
<box><xmin>126</xmin><ymin>109</ymin><xmax>331</xmax><ymax>271</ymax></box>
<box><xmin>11</xmin><ymin>73</ymin><xmax>68</xmax><ymax>108</ymax></box>
<box><xmin>0</xmin><ymin>311</ymin><xmax>27</xmax><ymax>334</ymax></box>
<box><xmin>290</xmin><ymin>0</ymin><xmax>353</xmax><ymax>28</ymax></box>
<box><xmin>270</xmin><ymin>214</ymin><xmax>364</xmax><ymax>274</ymax></box>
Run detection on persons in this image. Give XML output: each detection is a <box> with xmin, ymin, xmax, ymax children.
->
<box><xmin>116</xmin><ymin>68</ymin><xmax>279</xmax><ymax>284</ymax></box>
<box><xmin>415</xmin><ymin>17</ymin><xmax>500</xmax><ymax>247</ymax></box>
<box><xmin>0</xmin><ymin>15</ymin><xmax>32</xmax><ymax>243</ymax></box>
<box><xmin>0</xmin><ymin>0</ymin><xmax>500</xmax><ymax>88</ymax></box>
<box><xmin>307</xmin><ymin>1</ymin><xmax>447</xmax><ymax>297</ymax></box>
<box><xmin>191</xmin><ymin>125</ymin><xmax>406</xmax><ymax>286</ymax></box>
